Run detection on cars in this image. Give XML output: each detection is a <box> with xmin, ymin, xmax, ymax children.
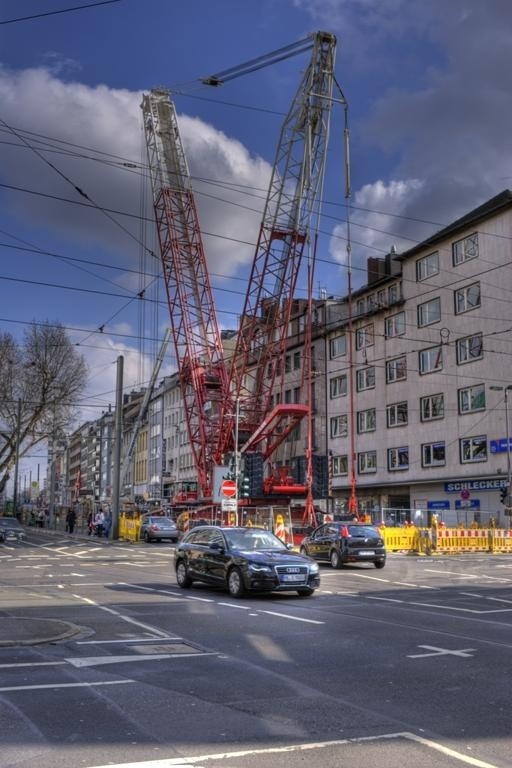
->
<box><xmin>300</xmin><ymin>521</ymin><xmax>386</xmax><ymax>568</ymax></box>
<box><xmin>0</xmin><ymin>516</ymin><xmax>25</xmax><ymax>544</ymax></box>
<box><xmin>173</xmin><ymin>526</ymin><xmax>320</xmax><ymax>598</ymax></box>
<box><xmin>140</xmin><ymin>516</ymin><xmax>178</xmax><ymax>542</ymax></box>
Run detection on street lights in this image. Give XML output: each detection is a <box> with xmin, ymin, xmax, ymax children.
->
<box><xmin>490</xmin><ymin>385</ymin><xmax>512</xmax><ymax>503</ymax></box>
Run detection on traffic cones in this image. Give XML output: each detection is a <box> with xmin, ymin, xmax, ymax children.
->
<box><xmin>229</xmin><ymin>514</ymin><xmax>234</xmax><ymax>527</ymax></box>
<box><xmin>181</xmin><ymin>513</ymin><xmax>190</xmax><ymax>532</ymax></box>
<box><xmin>275</xmin><ymin>515</ymin><xmax>285</xmax><ymax>543</ymax></box>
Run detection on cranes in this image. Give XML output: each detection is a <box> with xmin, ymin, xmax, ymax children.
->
<box><xmin>140</xmin><ymin>32</ymin><xmax>336</xmax><ymax>513</ymax></box>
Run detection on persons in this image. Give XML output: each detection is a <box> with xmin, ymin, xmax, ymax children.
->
<box><xmin>87</xmin><ymin>509</ymin><xmax>104</xmax><ymax>537</ymax></box>
<box><xmin>66</xmin><ymin>508</ymin><xmax>77</xmax><ymax>534</ymax></box>
<box><xmin>31</xmin><ymin>508</ymin><xmax>49</xmax><ymax>528</ymax></box>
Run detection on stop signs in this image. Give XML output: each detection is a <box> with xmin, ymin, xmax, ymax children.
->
<box><xmin>221</xmin><ymin>480</ymin><xmax>236</xmax><ymax>497</ymax></box>
